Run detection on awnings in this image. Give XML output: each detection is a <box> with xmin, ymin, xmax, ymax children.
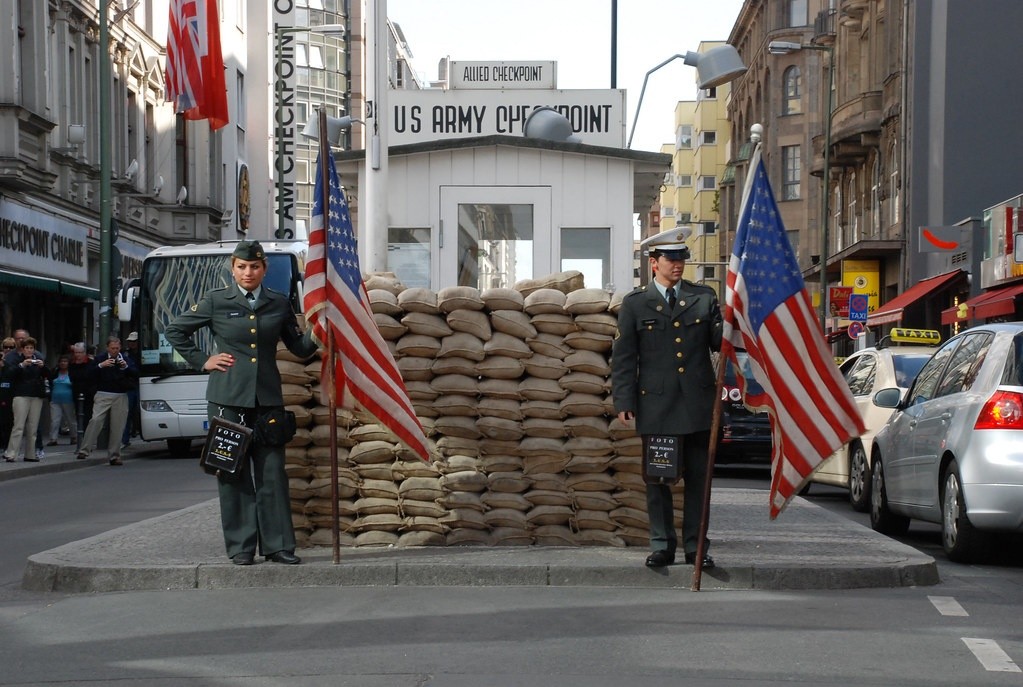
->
<box><xmin>942</xmin><ymin>285</ymin><xmax>1021</xmax><ymax>324</ymax></box>
<box><xmin>866</xmin><ymin>268</ymin><xmax>963</xmax><ymax>328</ymax></box>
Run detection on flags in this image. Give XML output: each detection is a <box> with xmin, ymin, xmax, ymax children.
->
<box><xmin>302</xmin><ymin>108</ymin><xmax>434</xmax><ymax>465</ymax></box>
<box><xmin>720</xmin><ymin>145</ymin><xmax>869</xmax><ymax>521</ymax></box>
<box><xmin>165</xmin><ymin>0</ymin><xmax>230</xmax><ymax>133</ymax></box>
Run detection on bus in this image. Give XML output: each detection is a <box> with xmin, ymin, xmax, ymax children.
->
<box><xmin>118</xmin><ymin>239</ymin><xmax>308</xmax><ymax>458</ymax></box>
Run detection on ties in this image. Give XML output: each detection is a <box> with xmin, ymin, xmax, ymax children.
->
<box><xmin>666</xmin><ymin>288</ymin><xmax>678</xmax><ymax>310</ymax></box>
<box><xmin>245</xmin><ymin>292</ymin><xmax>255</xmax><ymax>300</ymax></box>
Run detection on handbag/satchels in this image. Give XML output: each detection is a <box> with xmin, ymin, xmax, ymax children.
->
<box><xmin>254</xmin><ymin>410</ymin><xmax>296</xmax><ymax>446</ymax></box>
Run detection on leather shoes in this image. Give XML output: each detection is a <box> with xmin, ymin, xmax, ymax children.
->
<box><xmin>685</xmin><ymin>552</ymin><xmax>713</xmax><ymax>568</ymax></box>
<box><xmin>233</xmin><ymin>551</ymin><xmax>254</xmax><ymax>565</ymax></box>
<box><xmin>646</xmin><ymin>550</ymin><xmax>674</xmax><ymax>567</ymax></box>
<box><xmin>266</xmin><ymin>549</ymin><xmax>300</xmax><ymax>565</ymax></box>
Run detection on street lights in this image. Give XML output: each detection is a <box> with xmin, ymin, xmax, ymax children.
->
<box><xmin>677</xmin><ymin>220</ymin><xmax>706</xmax><ymax>285</ymax></box>
<box><xmin>769</xmin><ymin>41</ymin><xmax>834</xmax><ymax>336</ymax></box>
<box><xmin>278</xmin><ymin>23</ymin><xmax>345</xmax><ymax>240</ymax></box>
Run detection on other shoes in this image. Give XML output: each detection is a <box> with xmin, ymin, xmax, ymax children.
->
<box><xmin>3</xmin><ymin>454</ymin><xmax>15</xmax><ymax>462</ymax></box>
<box><xmin>77</xmin><ymin>454</ymin><xmax>85</xmax><ymax>459</ymax></box>
<box><xmin>23</xmin><ymin>456</ymin><xmax>40</xmax><ymax>462</ymax></box>
<box><xmin>110</xmin><ymin>458</ymin><xmax>123</xmax><ymax>466</ymax></box>
<box><xmin>47</xmin><ymin>440</ymin><xmax>57</xmax><ymax>446</ymax></box>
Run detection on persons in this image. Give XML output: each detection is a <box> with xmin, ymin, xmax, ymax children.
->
<box><xmin>611</xmin><ymin>226</ymin><xmax>723</xmax><ymax>568</ymax></box>
<box><xmin>0</xmin><ymin>329</ymin><xmax>145</xmax><ymax>466</ymax></box>
<box><xmin>166</xmin><ymin>243</ymin><xmax>319</xmax><ymax>567</ymax></box>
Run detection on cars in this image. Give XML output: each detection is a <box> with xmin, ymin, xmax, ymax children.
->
<box><xmin>871</xmin><ymin>322</ymin><xmax>1023</xmax><ymax>564</ymax></box>
<box><xmin>714</xmin><ymin>352</ymin><xmax>772</xmax><ymax>479</ymax></box>
<box><xmin>797</xmin><ymin>328</ymin><xmax>941</xmax><ymax>513</ymax></box>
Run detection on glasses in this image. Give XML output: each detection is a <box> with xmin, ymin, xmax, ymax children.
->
<box><xmin>3</xmin><ymin>346</ymin><xmax>15</xmax><ymax>350</ymax></box>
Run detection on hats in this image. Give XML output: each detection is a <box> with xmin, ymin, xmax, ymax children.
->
<box><xmin>127</xmin><ymin>331</ymin><xmax>138</xmax><ymax>341</ymax></box>
<box><xmin>233</xmin><ymin>241</ymin><xmax>264</xmax><ymax>262</ymax></box>
<box><xmin>640</xmin><ymin>228</ymin><xmax>694</xmax><ymax>259</ymax></box>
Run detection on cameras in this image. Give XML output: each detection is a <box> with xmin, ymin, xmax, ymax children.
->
<box><xmin>31</xmin><ymin>360</ymin><xmax>37</xmax><ymax>364</ymax></box>
